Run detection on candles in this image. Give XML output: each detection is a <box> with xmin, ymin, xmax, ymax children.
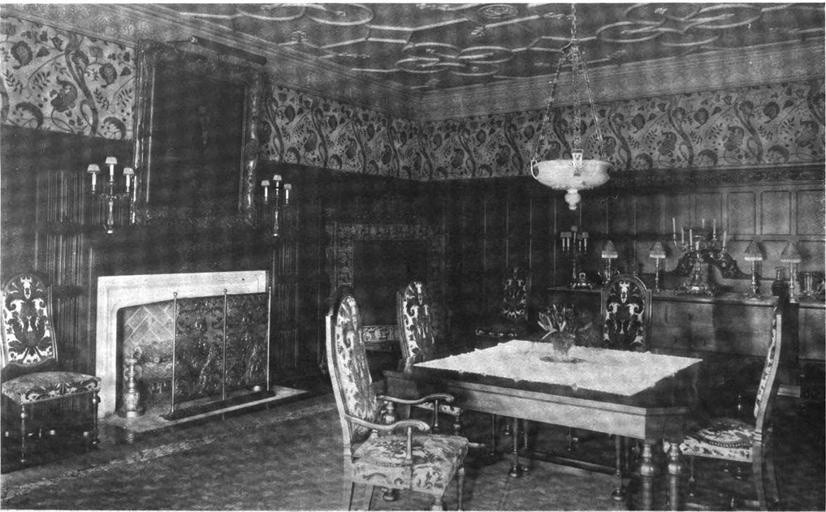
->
<box><xmin>672</xmin><ymin>217</ymin><xmax>727</xmax><ymax>251</ymax></box>
<box><xmin>561</xmin><ymin>237</ymin><xmax>588</xmax><ymax>253</ymax></box>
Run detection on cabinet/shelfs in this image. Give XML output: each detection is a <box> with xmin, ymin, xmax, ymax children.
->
<box><xmin>547</xmin><ymin>276</ymin><xmax>825</xmax><ymax>380</ymax></box>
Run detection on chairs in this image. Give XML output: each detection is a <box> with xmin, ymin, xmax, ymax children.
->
<box><xmin>565</xmin><ymin>279</ymin><xmax>657</xmax><ymax>460</ymax></box>
<box><xmin>0</xmin><ymin>268</ymin><xmax>100</xmax><ymax>463</ymax></box>
<box><xmin>390</xmin><ymin>275</ymin><xmax>487</xmax><ymax>470</ymax></box>
<box><xmin>661</xmin><ymin>297</ymin><xmax>790</xmax><ymax>506</ymax></box>
<box><xmin>325</xmin><ymin>288</ymin><xmax>466</xmax><ymax>507</ymax></box>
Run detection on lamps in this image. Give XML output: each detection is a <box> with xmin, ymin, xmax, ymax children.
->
<box><xmin>86</xmin><ymin>155</ymin><xmax>135</xmax><ymax>235</ymax></box>
<box><xmin>261</xmin><ymin>174</ymin><xmax>292</xmax><ymax>237</ymax></box>
<box><xmin>742</xmin><ymin>240</ymin><xmax>764</xmax><ymax>299</ymax></box>
<box><xmin>531</xmin><ymin>4</ymin><xmax>613</xmax><ymax>211</ymax></box>
<box><xmin>649</xmin><ymin>242</ymin><xmax>667</xmax><ymax>293</ymax></box>
<box><xmin>602</xmin><ymin>240</ymin><xmax>618</xmax><ymax>286</ymax></box>
<box><xmin>781</xmin><ymin>239</ymin><xmax>803</xmax><ymax>297</ymax></box>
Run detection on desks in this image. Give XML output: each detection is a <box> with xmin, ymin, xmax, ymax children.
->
<box><xmin>408</xmin><ymin>337</ymin><xmax>742</xmax><ymax>502</ymax></box>
